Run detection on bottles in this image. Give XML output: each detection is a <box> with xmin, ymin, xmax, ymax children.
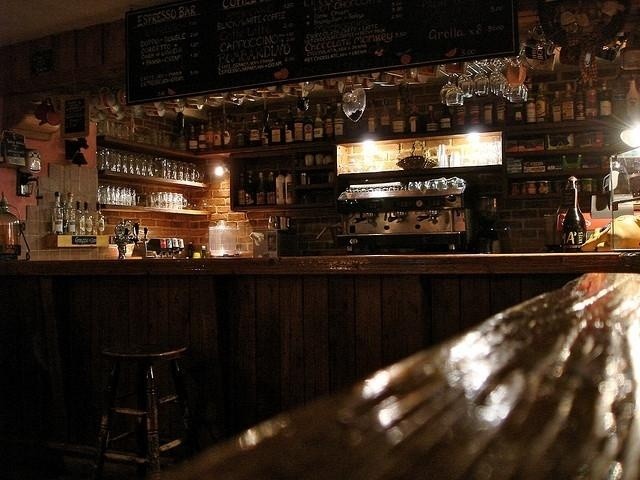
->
<box><xmin>236</xmin><ymin>76</ymin><xmax>639</xmax><ymax>207</ymax></box>
<box><xmin>0</xmin><ymin>193</ymin><xmax>21</xmax><ymax>257</ymax></box>
<box><xmin>50</xmin><ymin>192</ymin><xmax>105</xmax><ymax>235</ymax></box>
<box><xmin>201</xmin><ymin>246</ymin><xmax>207</xmax><ymax>253</ymax></box>
<box><xmin>96</xmin><ymin>119</ymin><xmax>172</xmax><ymax>148</ymax></box>
<box><xmin>100</xmin><ymin>148</ymin><xmax>154</xmax><ymax>175</ymax></box>
<box><xmin>268</xmin><ymin>215</ymin><xmax>292</xmax><ymax>229</ymax></box>
<box><xmin>188</xmin><ymin>242</ymin><xmax>194</xmax><ymax>257</ymax></box>
<box><xmin>174</xmin><ymin>108</ymin><xmax>236</xmax><ymax>150</ymax></box>
<box><xmin>160</xmin><ymin>238</ymin><xmax>185</xmax><ymax>248</ymax></box>
<box><xmin>559</xmin><ymin>176</ymin><xmax>588</xmax><ymax>252</ymax></box>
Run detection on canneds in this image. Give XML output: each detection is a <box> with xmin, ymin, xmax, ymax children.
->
<box><xmin>160</xmin><ymin>238</ymin><xmax>184</xmax><ymax>248</ymax></box>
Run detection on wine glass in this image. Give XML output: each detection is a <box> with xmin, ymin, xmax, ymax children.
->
<box><xmin>440</xmin><ymin>56</ymin><xmax>531</xmax><ymax>106</ymax></box>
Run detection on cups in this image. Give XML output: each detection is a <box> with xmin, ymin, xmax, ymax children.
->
<box><xmin>208</xmin><ymin>226</ymin><xmax>238</xmax><ymax>257</ymax></box>
<box><xmin>97</xmin><ymin>184</ymin><xmax>187</xmax><ymax>210</ymax></box>
<box><xmin>154</xmin><ymin>155</ymin><xmax>202</xmax><ymax>182</ymax></box>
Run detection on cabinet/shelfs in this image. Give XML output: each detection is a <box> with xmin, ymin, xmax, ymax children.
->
<box><xmin>94</xmin><ymin>128</ymin><xmax>210</xmax><ymax>221</ymax></box>
<box><xmin>193</xmin><ymin>117</ymin><xmax>615</xmax><ymax>209</ymax></box>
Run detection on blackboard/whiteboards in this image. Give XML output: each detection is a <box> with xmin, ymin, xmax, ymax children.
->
<box><xmin>125</xmin><ymin>0</ymin><xmax>519</xmax><ymax>104</ymax></box>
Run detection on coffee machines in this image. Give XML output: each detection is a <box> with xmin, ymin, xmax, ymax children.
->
<box><xmin>335</xmin><ymin>190</ymin><xmax>478</xmax><ymax>255</ymax></box>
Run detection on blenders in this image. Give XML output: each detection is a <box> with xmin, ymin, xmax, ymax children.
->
<box><xmin>476</xmin><ymin>198</ymin><xmax>503</xmax><ymax>255</ymax></box>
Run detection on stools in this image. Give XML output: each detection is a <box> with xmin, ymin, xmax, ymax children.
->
<box><xmin>89</xmin><ymin>342</ymin><xmax>192</xmax><ymax>480</ymax></box>
